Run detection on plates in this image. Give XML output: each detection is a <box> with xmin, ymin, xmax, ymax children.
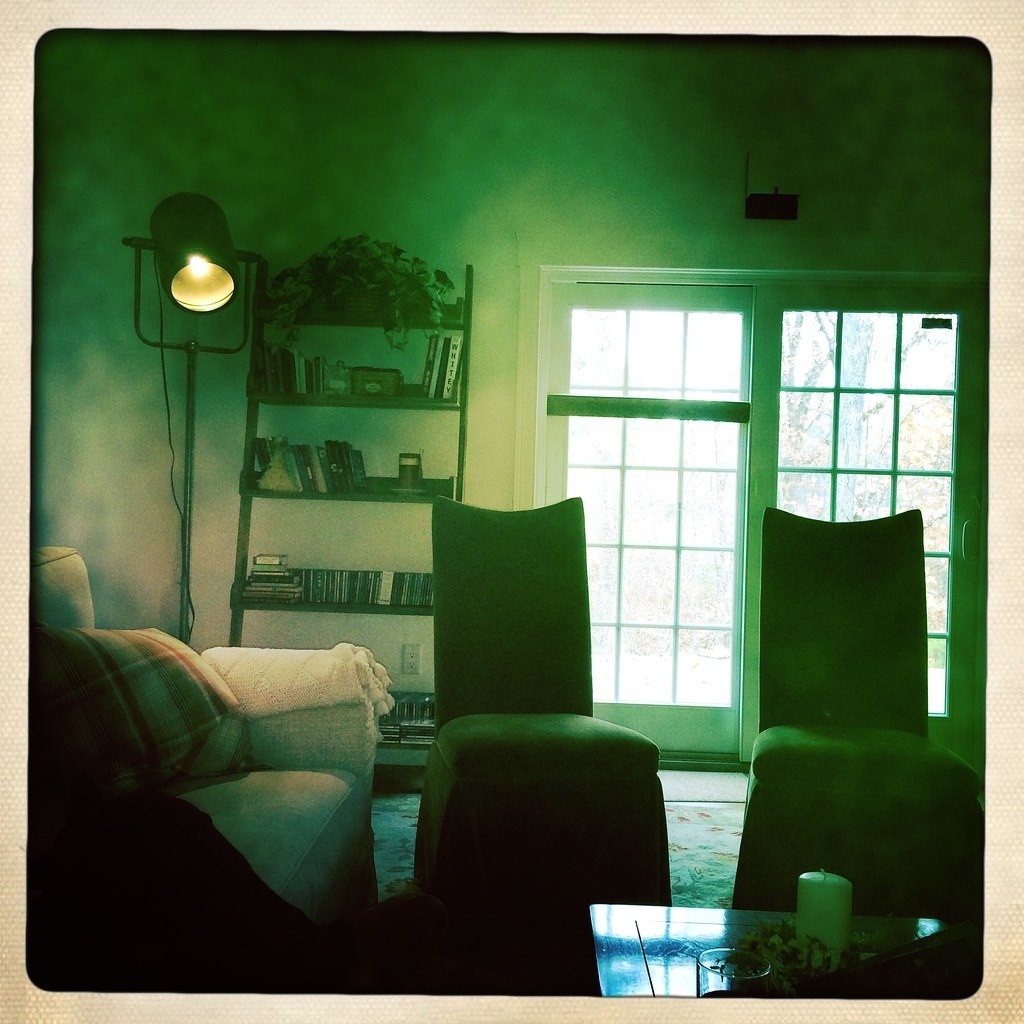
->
<box><xmin>389</xmin><ymin>488</ymin><xmax>423</xmax><ymax>495</ymax></box>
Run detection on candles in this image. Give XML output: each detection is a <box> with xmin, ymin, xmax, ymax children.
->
<box><xmin>798</xmin><ymin>868</ymin><xmax>853</xmax><ymax>951</ymax></box>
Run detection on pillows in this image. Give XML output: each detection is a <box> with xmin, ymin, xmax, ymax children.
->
<box><xmin>35</xmin><ymin>618</ymin><xmax>246</xmax><ymax>794</ymax></box>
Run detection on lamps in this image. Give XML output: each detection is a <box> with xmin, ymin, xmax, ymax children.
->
<box><xmin>122</xmin><ymin>190</ymin><xmax>259</xmax><ymax>645</ymax></box>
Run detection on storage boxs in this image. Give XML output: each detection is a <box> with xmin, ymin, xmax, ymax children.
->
<box><xmin>350</xmin><ymin>369</ymin><xmax>402</xmax><ymax>396</ymax></box>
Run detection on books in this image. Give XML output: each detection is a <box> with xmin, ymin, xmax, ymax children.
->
<box><xmin>242</xmin><ymin>556</ymin><xmax>434</xmax><ymax>606</ymax></box>
<box><xmin>255</xmin><ymin>435</ymin><xmax>425</xmax><ymax>494</ymax></box>
<box><xmin>257</xmin><ymin>330</ymin><xmax>462</xmax><ymax>399</ymax></box>
<box><xmin>379</xmin><ymin>695</ymin><xmax>435</xmax><ymax>745</ymax></box>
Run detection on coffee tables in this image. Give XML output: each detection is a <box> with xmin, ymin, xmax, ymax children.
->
<box><xmin>587</xmin><ymin>905</ymin><xmax>985</xmax><ymax>999</ymax></box>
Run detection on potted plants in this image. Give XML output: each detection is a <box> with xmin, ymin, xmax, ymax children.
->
<box><xmin>265</xmin><ymin>233</ymin><xmax>457</xmax><ymax>353</ymax></box>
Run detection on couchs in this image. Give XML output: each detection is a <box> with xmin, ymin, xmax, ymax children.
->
<box><xmin>34</xmin><ymin>543</ymin><xmax>381</xmax><ymax>926</ymax></box>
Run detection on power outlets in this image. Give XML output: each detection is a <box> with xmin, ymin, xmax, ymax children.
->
<box><xmin>401</xmin><ymin>643</ymin><xmax>422</xmax><ymax>675</ymax></box>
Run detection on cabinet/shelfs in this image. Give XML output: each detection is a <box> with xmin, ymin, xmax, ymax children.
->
<box><xmin>227</xmin><ymin>257</ymin><xmax>475</xmax><ymax>768</ymax></box>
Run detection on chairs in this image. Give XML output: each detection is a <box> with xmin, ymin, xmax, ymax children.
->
<box><xmin>730</xmin><ymin>507</ymin><xmax>983</xmax><ymax>922</ymax></box>
<box><xmin>416</xmin><ymin>494</ymin><xmax>671</xmax><ymax>941</ymax></box>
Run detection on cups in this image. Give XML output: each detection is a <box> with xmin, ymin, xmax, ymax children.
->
<box><xmin>699</xmin><ymin>949</ymin><xmax>770</xmax><ymax>998</ymax></box>
<box><xmin>399</xmin><ymin>453</ymin><xmax>421</xmax><ymax>488</ymax></box>
